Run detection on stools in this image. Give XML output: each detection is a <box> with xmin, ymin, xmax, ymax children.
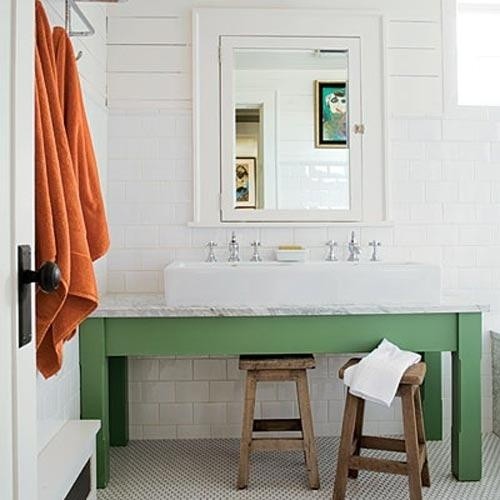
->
<box><xmin>332</xmin><ymin>355</ymin><xmax>430</xmax><ymax>500</ymax></box>
<box><xmin>238</xmin><ymin>353</ymin><xmax>321</xmax><ymax>491</ymax></box>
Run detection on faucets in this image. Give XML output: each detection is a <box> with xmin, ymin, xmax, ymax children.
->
<box><xmin>346</xmin><ymin>229</ymin><xmax>362</xmax><ymax>262</ymax></box>
<box><xmin>227</xmin><ymin>230</ymin><xmax>240</xmax><ymax>262</ymax></box>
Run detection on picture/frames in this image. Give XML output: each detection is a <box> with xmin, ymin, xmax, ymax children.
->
<box><xmin>313</xmin><ymin>79</ymin><xmax>348</xmax><ymax>149</ymax></box>
<box><xmin>236</xmin><ymin>156</ymin><xmax>257</xmax><ymax>208</ymax></box>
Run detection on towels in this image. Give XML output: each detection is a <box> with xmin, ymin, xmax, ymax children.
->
<box><xmin>343</xmin><ymin>337</ymin><xmax>422</xmax><ymax>409</ymax></box>
<box><xmin>35</xmin><ymin>0</ymin><xmax>111</xmax><ymax>380</ymax></box>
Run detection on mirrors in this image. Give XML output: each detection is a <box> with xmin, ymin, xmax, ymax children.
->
<box><xmin>218</xmin><ymin>33</ymin><xmax>364</xmax><ymax>223</ymax></box>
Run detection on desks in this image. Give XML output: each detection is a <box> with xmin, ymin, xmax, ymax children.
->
<box><xmin>78</xmin><ymin>293</ymin><xmax>492</xmax><ymax>489</ymax></box>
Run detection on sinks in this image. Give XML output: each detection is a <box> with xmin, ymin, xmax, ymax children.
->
<box><xmin>165</xmin><ymin>259</ymin><xmax>440</xmax><ymax>307</ymax></box>
